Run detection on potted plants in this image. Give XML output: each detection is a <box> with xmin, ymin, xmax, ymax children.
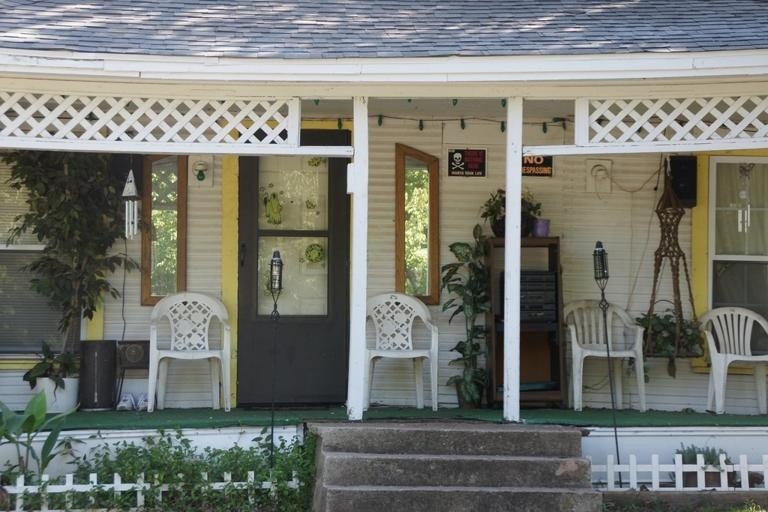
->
<box><xmin>477</xmin><ymin>186</ymin><xmax>542</xmax><ymax>238</ymax></box>
<box><xmin>0</xmin><ymin>149</ymin><xmax>146</xmax><ymax>414</ymax></box>
<box><xmin>673</xmin><ymin>443</ymin><xmax>735</xmax><ymax>487</ymax></box>
<box><xmin>439</xmin><ymin>224</ymin><xmax>492</xmax><ymax>410</ymax></box>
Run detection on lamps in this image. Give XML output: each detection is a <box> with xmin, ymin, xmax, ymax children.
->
<box><xmin>188</xmin><ymin>156</ymin><xmax>214</xmax><ymax>187</ymax></box>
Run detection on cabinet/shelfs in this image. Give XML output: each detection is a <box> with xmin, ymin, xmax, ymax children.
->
<box><xmin>484</xmin><ymin>236</ymin><xmax>567</xmax><ymax>411</ymax></box>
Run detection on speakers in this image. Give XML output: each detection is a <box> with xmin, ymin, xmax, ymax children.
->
<box><xmin>664</xmin><ymin>155</ymin><xmax>698</xmax><ymax>208</ymax></box>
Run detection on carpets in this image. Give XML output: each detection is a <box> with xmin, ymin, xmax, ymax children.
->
<box><xmin>6</xmin><ymin>407</ymin><xmax>768</xmax><ymax>431</ymax></box>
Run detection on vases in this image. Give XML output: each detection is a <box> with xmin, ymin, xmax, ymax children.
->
<box><xmin>79</xmin><ymin>340</ymin><xmax>116</xmax><ymax>409</ymax></box>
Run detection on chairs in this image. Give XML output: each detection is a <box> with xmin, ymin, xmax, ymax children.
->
<box><xmin>147</xmin><ymin>291</ymin><xmax>231</xmax><ymax>412</ymax></box>
<box><xmin>363</xmin><ymin>292</ymin><xmax>440</xmax><ymax>412</ymax></box>
<box><xmin>699</xmin><ymin>306</ymin><xmax>768</xmax><ymax>415</ymax></box>
<box><xmin>563</xmin><ymin>299</ymin><xmax>646</xmax><ymax>413</ymax></box>
